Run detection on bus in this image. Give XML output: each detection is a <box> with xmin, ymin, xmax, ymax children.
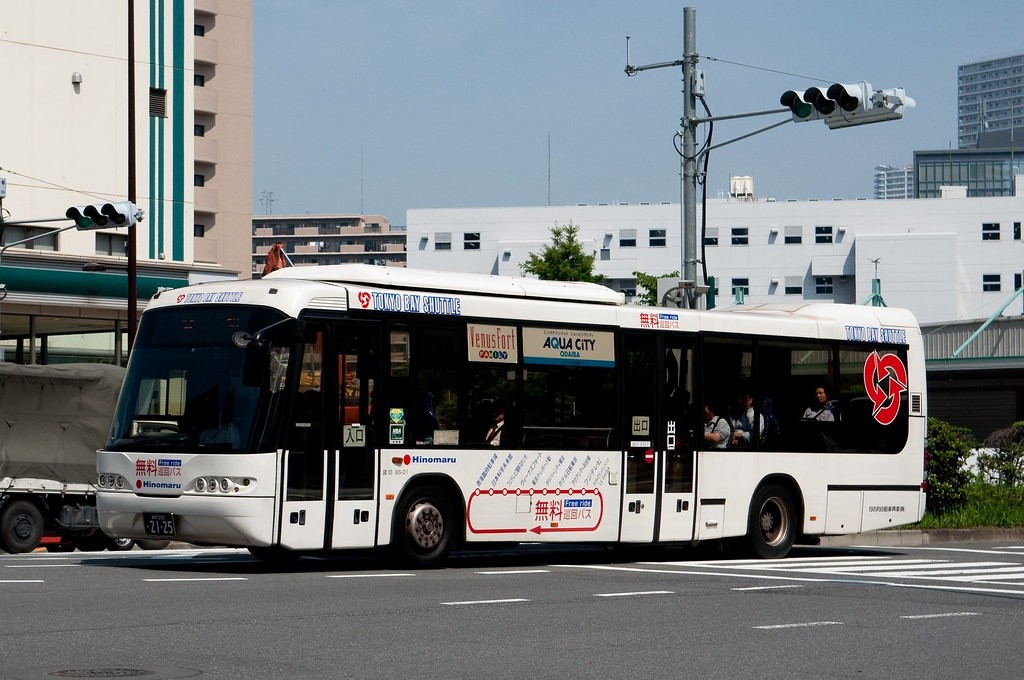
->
<box><xmin>96</xmin><ymin>265</ymin><xmax>927</xmax><ymax>566</ymax></box>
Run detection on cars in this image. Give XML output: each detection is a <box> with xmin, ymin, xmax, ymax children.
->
<box><xmin>299</xmin><ymin>361</ymin><xmax>321</xmax><ymax>387</ymax></box>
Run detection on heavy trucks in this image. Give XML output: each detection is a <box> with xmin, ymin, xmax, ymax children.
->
<box><xmin>0</xmin><ymin>361</ymin><xmax>170</xmax><ymax>554</ymax></box>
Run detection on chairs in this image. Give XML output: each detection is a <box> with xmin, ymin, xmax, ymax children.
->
<box><xmin>757</xmin><ymin>396</ymin><xmax>780</xmax><ymax>436</ymax></box>
<box><xmin>377</xmin><ymin>371</ymin><xmax>435</xmax><ymax>443</ymax></box>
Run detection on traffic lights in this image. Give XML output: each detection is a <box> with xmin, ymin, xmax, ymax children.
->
<box><xmin>824</xmin><ymin>87</ymin><xmax>916</xmax><ymax>130</ymax></box>
<box><xmin>780</xmin><ymin>80</ymin><xmax>873</xmax><ymax>123</ymax></box>
<box><xmin>66</xmin><ymin>201</ymin><xmax>137</xmax><ymax>231</ymax></box>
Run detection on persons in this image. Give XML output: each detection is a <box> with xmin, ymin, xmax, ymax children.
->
<box><xmin>802</xmin><ymin>387</ymin><xmax>842</xmax><ymax>422</ymax></box>
<box><xmin>200</xmin><ymin>423</ymin><xmax>239</xmax><ymax>449</ymax></box>
<box><xmin>484</xmin><ymin>400</ymin><xmax>506</xmax><ymax>446</ymax></box>
<box><xmin>730</xmin><ymin>391</ymin><xmax>764</xmax><ymax>444</ymax></box>
<box><xmin>703</xmin><ymin>401</ymin><xmax>730</xmax><ymax>448</ymax></box>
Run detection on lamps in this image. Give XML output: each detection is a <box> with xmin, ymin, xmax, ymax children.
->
<box><xmin>771</xmin><ymin>278</ymin><xmax>778</xmax><ymax>284</ymax></box>
<box><xmin>605</xmin><ymin>231</ymin><xmax>613</xmax><ymax>237</ymax></box>
<box><xmin>504</xmin><ymin>249</ymin><xmax>512</xmax><ymax>256</ymax></box>
<box><xmin>421</xmin><ymin>232</ymin><xmax>429</xmax><ymax>240</ymax></box>
<box><xmin>72</xmin><ymin>72</ymin><xmax>81</xmax><ymax>83</ymax></box>
<box><xmin>772</xmin><ymin>228</ymin><xmax>778</xmax><ymax>233</ymax></box>
<box><xmin>839</xmin><ymin>226</ymin><xmax>846</xmax><ymax>232</ymax></box>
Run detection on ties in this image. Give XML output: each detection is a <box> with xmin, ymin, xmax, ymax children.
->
<box><xmin>487</xmin><ymin>424</ymin><xmax>498</xmax><ymax>441</ymax></box>
